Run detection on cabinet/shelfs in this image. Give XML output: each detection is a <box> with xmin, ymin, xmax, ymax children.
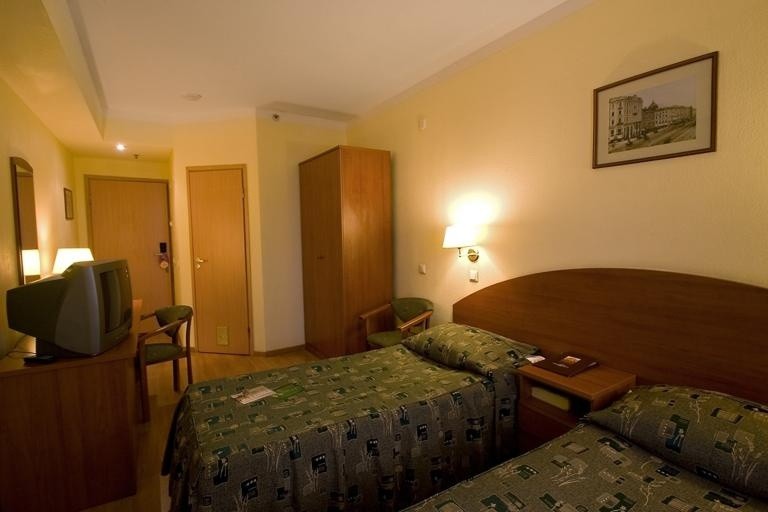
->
<box><xmin>299</xmin><ymin>146</ymin><xmax>391</xmax><ymax>359</ymax></box>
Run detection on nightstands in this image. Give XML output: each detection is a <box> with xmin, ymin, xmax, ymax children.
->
<box><xmin>515</xmin><ymin>364</ymin><xmax>636</xmax><ymax>452</ymax></box>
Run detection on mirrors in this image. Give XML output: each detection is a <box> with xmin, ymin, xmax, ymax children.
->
<box><xmin>10</xmin><ymin>156</ymin><xmax>39</xmax><ymax>285</ymax></box>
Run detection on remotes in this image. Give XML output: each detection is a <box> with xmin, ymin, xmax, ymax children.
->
<box><xmin>22</xmin><ymin>354</ymin><xmax>54</xmax><ymax>365</ymax></box>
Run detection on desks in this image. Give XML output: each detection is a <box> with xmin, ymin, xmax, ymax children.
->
<box><xmin>1</xmin><ymin>296</ymin><xmax>144</xmax><ymax>511</ymax></box>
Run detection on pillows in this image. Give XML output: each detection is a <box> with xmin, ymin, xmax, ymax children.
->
<box><xmin>584</xmin><ymin>384</ymin><xmax>768</xmax><ymax>498</ymax></box>
<box><xmin>402</xmin><ymin>321</ymin><xmax>537</xmax><ymax>374</ymax></box>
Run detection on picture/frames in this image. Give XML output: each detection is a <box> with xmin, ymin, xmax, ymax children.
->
<box><xmin>593</xmin><ymin>51</ymin><xmax>719</xmax><ymax>170</ymax></box>
<box><xmin>65</xmin><ymin>188</ymin><xmax>74</xmax><ymax>219</ymax></box>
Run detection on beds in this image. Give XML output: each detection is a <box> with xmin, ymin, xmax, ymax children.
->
<box><xmin>397</xmin><ymin>424</ymin><xmax>767</xmax><ymax>511</ymax></box>
<box><xmin>161</xmin><ymin>341</ymin><xmax>511</xmax><ymax>509</ymax></box>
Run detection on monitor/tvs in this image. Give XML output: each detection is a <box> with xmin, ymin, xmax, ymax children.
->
<box><xmin>5</xmin><ymin>257</ymin><xmax>134</xmax><ymax>354</ymax></box>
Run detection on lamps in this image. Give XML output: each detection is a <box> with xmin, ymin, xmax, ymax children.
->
<box><xmin>442</xmin><ymin>224</ymin><xmax>484</xmax><ymax>264</ymax></box>
<box><xmin>53</xmin><ymin>248</ymin><xmax>93</xmax><ymax>274</ymax></box>
<box><xmin>22</xmin><ymin>249</ymin><xmax>40</xmax><ymax>284</ymax></box>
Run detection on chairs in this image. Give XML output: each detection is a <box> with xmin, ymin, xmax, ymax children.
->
<box><xmin>137</xmin><ymin>306</ymin><xmax>194</xmax><ymax>422</ymax></box>
<box><xmin>359</xmin><ymin>298</ymin><xmax>434</xmax><ymax>352</ymax></box>
